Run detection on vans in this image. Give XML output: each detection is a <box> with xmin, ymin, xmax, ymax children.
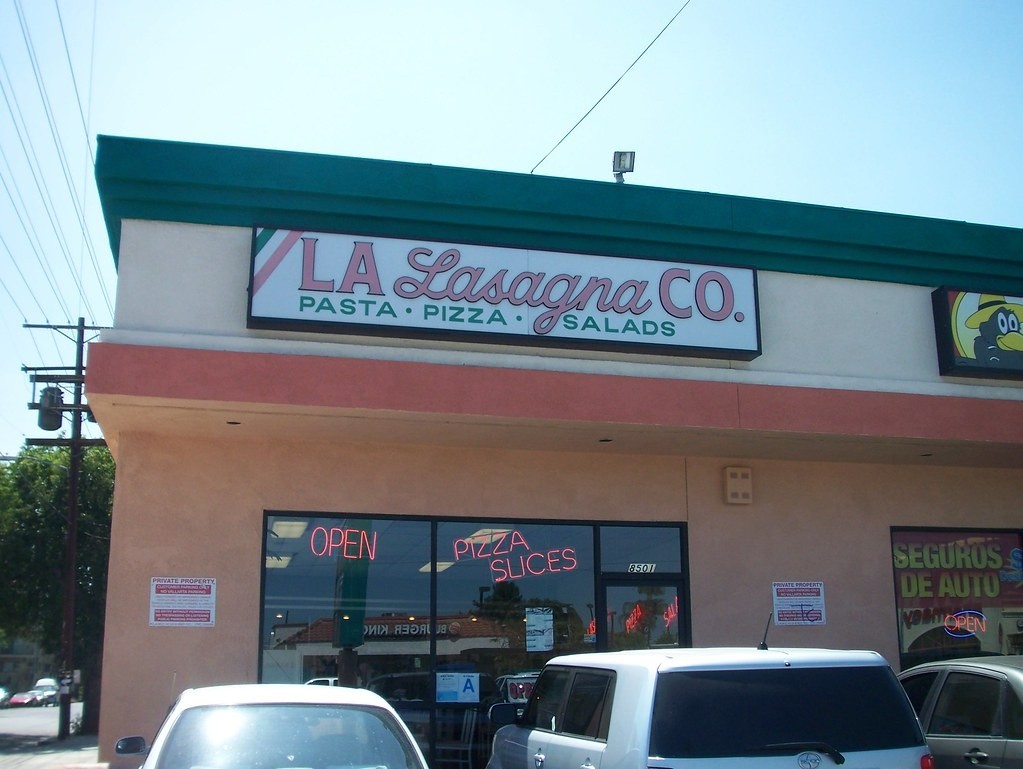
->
<box><xmin>34</xmin><ymin>676</ymin><xmax>59</xmax><ymax>707</ymax></box>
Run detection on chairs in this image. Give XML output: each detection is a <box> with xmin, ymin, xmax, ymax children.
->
<box><xmin>434</xmin><ymin>706</ymin><xmax>479</xmax><ymax>769</ymax></box>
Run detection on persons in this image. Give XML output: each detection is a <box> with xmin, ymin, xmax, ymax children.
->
<box><xmin>366</xmin><ymin>670</ymin><xmax>389</xmax><ymax>698</ymax></box>
<box><xmin>316</xmin><ymin>655</ymin><xmax>337</xmax><ymax>682</ymax></box>
<box><xmin>338</xmin><ymin>656</ymin><xmax>358</xmax><ymax>687</ymax></box>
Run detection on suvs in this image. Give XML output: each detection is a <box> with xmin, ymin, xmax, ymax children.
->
<box><xmin>484</xmin><ymin>643</ymin><xmax>936</xmax><ymax>768</ymax></box>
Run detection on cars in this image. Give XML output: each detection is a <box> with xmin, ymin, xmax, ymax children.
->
<box><xmin>112</xmin><ymin>683</ymin><xmax>429</xmax><ymax>768</ymax></box>
<box><xmin>302</xmin><ymin>658</ymin><xmax>547</xmax><ymax>747</ymax></box>
<box><xmin>0</xmin><ymin>688</ymin><xmax>46</xmax><ymax>710</ymax></box>
<box><xmin>887</xmin><ymin>655</ymin><xmax>1023</xmax><ymax>768</ymax></box>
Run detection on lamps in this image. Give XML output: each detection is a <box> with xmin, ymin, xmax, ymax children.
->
<box><xmin>612</xmin><ymin>151</ymin><xmax>635</xmax><ymax>184</ymax></box>
